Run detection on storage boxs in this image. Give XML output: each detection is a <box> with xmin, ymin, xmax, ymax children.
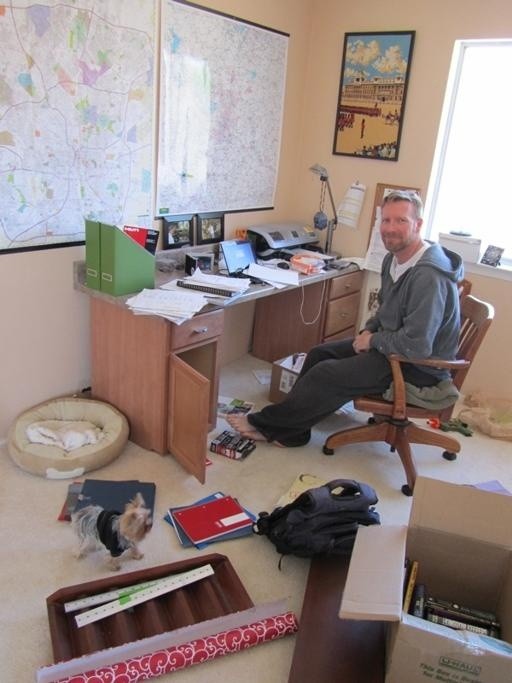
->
<box><xmin>336</xmin><ymin>473</ymin><xmax>512</xmax><ymax>683</ymax></box>
<box><xmin>269</xmin><ymin>351</ymin><xmax>307</xmax><ymax>401</ymax></box>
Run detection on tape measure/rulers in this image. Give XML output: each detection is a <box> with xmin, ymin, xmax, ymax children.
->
<box><xmin>64</xmin><ymin>564</ymin><xmax>216</xmax><ymax>629</ymax></box>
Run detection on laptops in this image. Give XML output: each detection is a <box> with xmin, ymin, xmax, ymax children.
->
<box><xmin>219</xmin><ymin>240</ymin><xmax>262</xmax><ymax>283</ymax></box>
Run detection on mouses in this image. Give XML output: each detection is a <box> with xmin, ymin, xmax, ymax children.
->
<box><xmin>277</xmin><ymin>262</ymin><xmax>289</xmax><ymax>270</ymax></box>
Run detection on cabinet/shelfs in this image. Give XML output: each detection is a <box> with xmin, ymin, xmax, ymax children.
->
<box><xmin>90</xmin><ymin>296</ymin><xmax>226</xmax><ymax>484</ymax></box>
<box><xmin>252</xmin><ymin>270</ymin><xmax>366</xmax><ymax>363</ymax></box>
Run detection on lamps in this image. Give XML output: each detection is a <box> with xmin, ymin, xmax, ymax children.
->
<box><xmin>309</xmin><ymin>162</ymin><xmax>338</xmax><ymax>258</ymax></box>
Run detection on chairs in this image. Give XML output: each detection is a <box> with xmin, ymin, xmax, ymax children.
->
<box><xmin>323</xmin><ymin>276</ymin><xmax>494</xmax><ymax>496</ymax></box>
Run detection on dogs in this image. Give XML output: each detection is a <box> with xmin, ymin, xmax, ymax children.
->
<box><xmin>69</xmin><ymin>492</ymin><xmax>153</xmax><ymax>572</ymax></box>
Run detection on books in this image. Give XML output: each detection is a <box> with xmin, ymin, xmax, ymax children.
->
<box><xmin>60</xmin><ymin>476</ymin><xmax>259</xmax><ymax>550</ymax></box>
<box><xmin>125</xmin><ymin>266</ymin><xmax>252</xmax><ymax>327</ymax></box>
<box><xmin>217</xmin><ymin>394</ymin><xmax>255</xmax><ymax>419</ymax></box>
<box><xmin>252</xmin><ymin>368</ymin><xmax>272</xmax><ymax>385</ymax></box>
<box><xmin>124</xmin><ymin>223</ymin><xmax>160</xmax><ymax>255</ymax></box>
<box><xmin>404</xmin><ymin>555</ymin><xmax>505</xmax><ymax>642</ymax></box>
<box><xmin>210</xmin><ymin>429</ymin><xmax>257</xmax><ymax>462</ymax></box>
<box><xmin>242</xmin><ymin>260</ymin><xmax>301</xmax><ymax>291</ymax></box>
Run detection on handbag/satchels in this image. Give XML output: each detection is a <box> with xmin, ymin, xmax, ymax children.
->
<box><xmin>261</xmin><ymin>478</ymin><xmax>381</xmax><ymax>559</ymax></box>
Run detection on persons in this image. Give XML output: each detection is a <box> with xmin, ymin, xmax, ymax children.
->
<box><xmin>169</xmin><ymin>222</ymin><xmax>179</xmax><ymax>245</ymax></box>
<box><xmin>247</xmin><ymin>187</ymin><xmax>465</xmax><ymax>447</ymax></box>
<box><xmin>207</xmin><ymin>222</ymin><xmax>215</xmax><ymax>238</ymax></box>
<box><xmin>218</xmin><ymin>402</ymin><xmax>251</xmax><ymax>415</ymax></box>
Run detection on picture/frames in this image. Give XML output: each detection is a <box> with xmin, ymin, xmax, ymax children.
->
<box><xmin>331</xmin><ymin>32</ymin><xmax>416</xmax><ymax>162</ymax></box>
<box><xmin>163</xmin><ymin>213</ymin><xmax>224</xmax><ymax>250</ymax></box>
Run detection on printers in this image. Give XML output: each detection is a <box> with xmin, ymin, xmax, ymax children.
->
<box><xmin>244</xmin><ymin>222</ymin><xmax>334</xmax><ymax>262</ymax></box>
